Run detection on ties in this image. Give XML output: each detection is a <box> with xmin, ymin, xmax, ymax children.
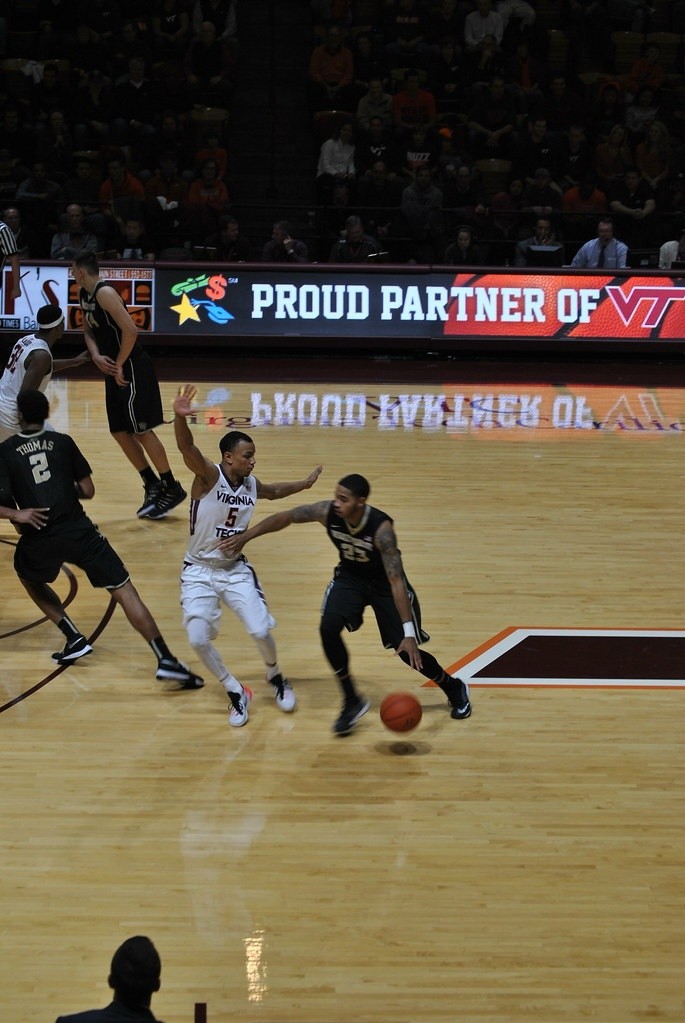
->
<box><xmin>596</xmin><ymin>246</ymin><xmax>606</xmax><ymax>265</ymax></box>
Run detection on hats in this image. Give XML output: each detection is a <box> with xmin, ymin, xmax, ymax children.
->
<box><xmin>535</xmin><ymin>168</ymin><xmax>549</xmax><ymax>177</ymax></box>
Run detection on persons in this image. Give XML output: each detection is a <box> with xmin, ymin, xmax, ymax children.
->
<box><xmin>0</xmin><ymin>0</ymin><xmax>685</xmax><ymax>271</ymax></box>
<box><xmin>0</xmin><ymin>304</ymin><xmax>92</xmax><ymax>436</ymax></box>
<box><xmin>0</xmin><ymin>221</ymin><xmax>22</xmax><ymax>300</ymax></box>
<box><xmin>173</xmin><ymin>383</ymin><xmax>323</xmax><ymax>727</ymax></box>
<box><xmin>71</xmin><ymin>249</ymin><xmax>187</xmax><ymax>518</ymax></box>
<box><xmin>0</xmin><ymin>390</ymin><xmax>204</xmax><ymax>687</ymax></box>
<box><xmin>218</xmin><ymin>474</ymin><xmax>472</xmax><ymax>732</ymax></box>
<box><xmin>56</xmin><ymin>936</ymin><xmax>162</xmax><ymax>1023</ymax></box>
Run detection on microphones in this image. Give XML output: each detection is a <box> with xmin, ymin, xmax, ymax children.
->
<box><xmin>605</xmin><ymin>237</ymin><xmax>610</xmax><ymax>242</ymax></box>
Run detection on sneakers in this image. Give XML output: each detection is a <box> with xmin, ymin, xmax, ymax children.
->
<box><xmin>148</xmin><ymin>480</ymin><xmax>187</xmax><ymax>518</ymax></box>
<box><xmin>264</xmin><ymin>669</ymin><xmax>295</xmax><ymax>712</ymax></box>
<box><xmin>447</xmin><ymin>677</ymin><xmax>471</xmax><ymax>719</ymax></box>
<box><xmin>137</xmin><ymin>481</ymin><xmax>162</xmax><ymax>516</ymax></box>
<box><xmin>227</xmin><ymin>682</ymin><xmax>253</xmax><ymax>727</ymax></box>
<box><xmin>50</xmin><ymin>635</ymin><xmax>93</xmax><ymax>662</ymax></box>
<box><xmin>334</xmin><ymin>699</ymin><xmax>369</xmax><ymax>738</ymax></box>
<box><xmin>155</xmin><ymin>658</ymin><xmax>203</xmax><ymax>688</ymax></box>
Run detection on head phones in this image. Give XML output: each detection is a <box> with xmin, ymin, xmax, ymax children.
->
<box><xmin>455</xmin><ymin>225</ymin><xmax>478</xmax><ymax>240</ymax></box>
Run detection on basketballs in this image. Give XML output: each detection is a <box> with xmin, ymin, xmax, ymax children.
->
<box><xmin>380</xmin><ymin>691</ymin><xmax>423</xmax><ymax>733</ymax></box>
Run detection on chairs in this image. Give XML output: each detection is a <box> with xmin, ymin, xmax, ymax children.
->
<box><xmin>2</xmin><ymin>7</ymin><xmax>685</xmax><ymax>237</ymax></box>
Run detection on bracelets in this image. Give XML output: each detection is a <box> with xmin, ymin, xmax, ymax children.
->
<box><xmin>403</xmin><ymin>621</ymin><xmax>416</xmax><ymax>638</ymax></box>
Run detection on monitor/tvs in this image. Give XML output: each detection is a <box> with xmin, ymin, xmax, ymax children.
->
<box><xmin>626</xmin><ymin>249</ymin><xmax>659</xmax><ymax>269</ymax></box>
<box><xmin>671</xmin><ymin>261</ymin><xmax>685</xmax><ymax>269</ymax></box>
<box><xmin>471</xmin><ymin>240</ymin><xmax>502</xmax><ymax>266</ymax></box>
<box><xmin>376</xmin><ymin>238</ymin><xmax>414</xmax><ymax>264</ymax></box>
<box><xmin>194</xmin><ymin>246</ymin><xmax>216</xmax><ymax>262</ymax></box>
<box><xmin>529</xmin><ymin>246</ymin><xmax>566</xmax><ymax>268</ymax></box>
